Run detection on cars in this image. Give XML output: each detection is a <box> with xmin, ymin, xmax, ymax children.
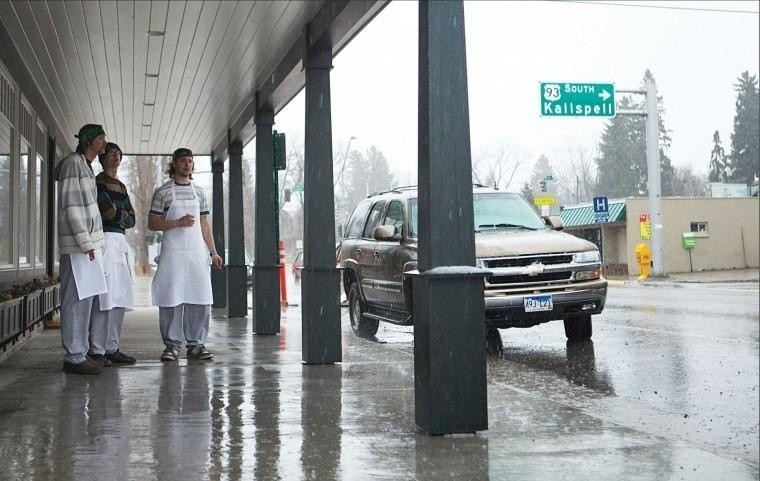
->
<box><xmin>291</xmin><ymin>251</ymin><xmax>303</xmax><ymax>282</ymax></box>
<box><xmin>207</xmin><ymin>249</ymin><xmax>255</xmax><ymax>288</ymax></box>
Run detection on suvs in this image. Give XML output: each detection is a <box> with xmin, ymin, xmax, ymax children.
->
<box><xmin>337</xmin><ymin>185</ymin><xmax>608</xmax><ymax>345</ymax></box>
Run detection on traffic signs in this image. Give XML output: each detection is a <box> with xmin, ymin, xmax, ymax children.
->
<box><xmin>541</xmin><ymin>82</ymin><xmax>616</xmax><ymax>118</ymax></box>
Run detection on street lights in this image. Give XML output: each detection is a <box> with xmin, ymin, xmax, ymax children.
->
<box><xmin>341</xmin><ymin>136</ymin><xmax>356</xmax><ymax>237</ymax></box>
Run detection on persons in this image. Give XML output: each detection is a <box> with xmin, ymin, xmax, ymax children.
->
<box><xmin>146</xmin><ymin>149</ymin><xmax>223</xmax><ymax>360</ymax></box>
<box><xmin>87</xmin><ymin>142</ymin><xmax>136</xmax><ymax>367</ymax></box>
<box><xmin>53</xmin><ymin>125</ymin><xmax>108</xmax><ymax>375</ymax></box>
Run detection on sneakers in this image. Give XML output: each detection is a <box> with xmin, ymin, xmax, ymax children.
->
<box><xmin>61</xmin><ymin>343</ymin><xmax>135</xmax><ymax>375</ymax></box>
<box><xmin>186</xmin><ymin>344</ymin><xmax>213</xmax><ymax>359</ymax></box>
<box><xmin>160</xmin><ymin>343</ymin><xmax>181</xmax><ymax>360</ymax></box>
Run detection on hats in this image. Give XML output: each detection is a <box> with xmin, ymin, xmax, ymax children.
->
<box><xmin>98</xmin><ymin>142</ymin><xmax>122</xmax><ymax>164</ymax></box>
<box><xmin>173</xmin><ymin>148</ymin><xmax>193</xmax><ymax>162</ymax></box>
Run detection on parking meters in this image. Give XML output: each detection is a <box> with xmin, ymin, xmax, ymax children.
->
<box><xmin>634</xmin><ymin>242</ymin><xmax>649</xmax><ymax>275</ymax></box>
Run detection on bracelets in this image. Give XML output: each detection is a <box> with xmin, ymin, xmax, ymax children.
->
<box><xmin>210</xmin><ymin>251</ymin><xmax>217</xmax><ymax>257</ymax></box>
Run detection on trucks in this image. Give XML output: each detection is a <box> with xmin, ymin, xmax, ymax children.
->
<box><xmin>701</xmin><ymin>183</ymin><xmax>760</xmax><ymax>197</ymax></box>
